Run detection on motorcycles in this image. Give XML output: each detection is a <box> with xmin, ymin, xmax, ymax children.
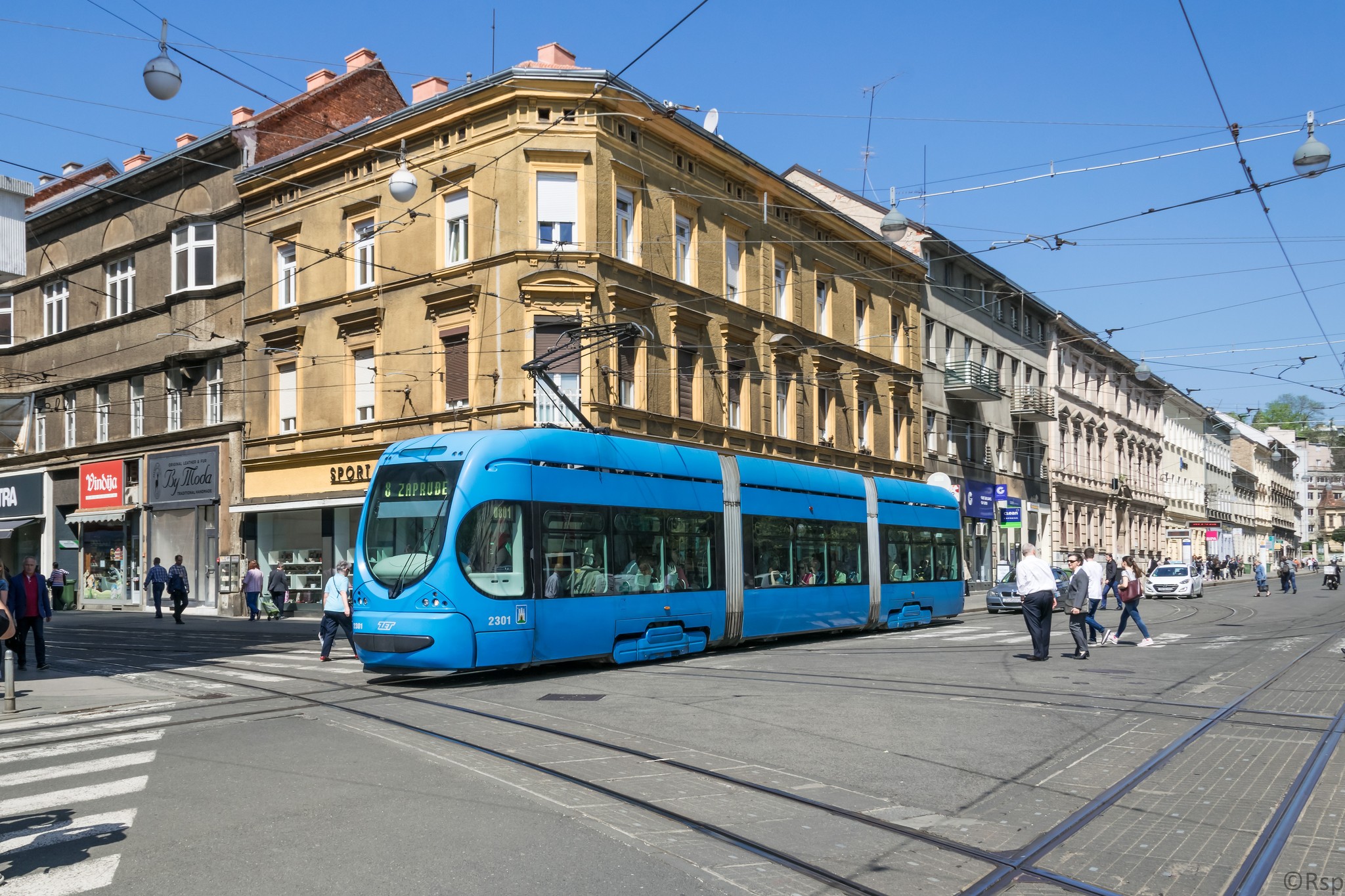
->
<box><xmin>1326</xmin><ymin>567</ymin><xmax>1344</xmax><ymax>590</ymax></box>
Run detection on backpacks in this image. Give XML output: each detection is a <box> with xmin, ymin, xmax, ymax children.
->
<box><xmin>1280</xmin><ymin>561</ymin><xmax>1289</xmax><ymax>573</ymax></box>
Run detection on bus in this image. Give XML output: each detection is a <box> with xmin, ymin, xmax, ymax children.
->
<box><xmin>353</xmin><ymin>316</ymin><xmax>965</xmax><ymax>673</ymax></box>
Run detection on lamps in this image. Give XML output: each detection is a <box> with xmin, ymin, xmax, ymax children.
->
<box><xmin>1271</xmin><ymin>443</ymin><xmax>1281</xmax><ymax>461</ymax></box>
<box><xmin>143</xmin><ymin>19</ymin><xmax>184</xmax><ymax>100</ymax></box>
<box><xmin>1229</xmin><ymin>425</ymin><xmax>1241</xmax><ymax>439</ymax></box>
<box><xmin>1293</xmin><ymin>110</ymin><xmax>1331</xmax><ymax>179</ymax></box>
<box><xmin>880</xmin><ymin>187</ymin><xmax>908</xmax><ymax>242</ymax></box>
<box><xmin>1135</xmin><ymin>351</ymin><xmax>1151</xmax><ymax>381</ymax></box>
<box><xmin>1301</xmin><ymin>468</ymin><xmax>1332</xmax><ymax>491</ymax></box>
<box><xmin>389</xmin><ymin>139</ymin><xmax>417</xmax><ymax>202</ymax></box>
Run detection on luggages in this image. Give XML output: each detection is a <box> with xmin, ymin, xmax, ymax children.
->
<box><xmin>259</xmin><ymin>596</ymin><xmax>281</xmax><ymax>620</ymax></box>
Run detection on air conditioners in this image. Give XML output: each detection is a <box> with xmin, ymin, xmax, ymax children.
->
<box><xmin>966</xmin><ymin>523</ymin><xmax>972</xmax><ymax>536</ymax></box>
<box><xmin>976</xmin><ymin>522</ymin><xmax>988</xmax><ymax>537</ymax></box>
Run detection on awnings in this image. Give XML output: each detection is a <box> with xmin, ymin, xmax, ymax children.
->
<box><xmin>65</xmin><ymin>508</ymin><xmax>133</xmax><ymax>524</ymax></box>
<box><xmin>0</xmin><ymin>519</ymin><xmax>35</xmax><ymax>539</ymax></box>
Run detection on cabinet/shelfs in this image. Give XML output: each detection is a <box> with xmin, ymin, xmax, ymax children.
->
<box><xmin>347</xmin><ymin>546</ymin><xmax>395</xmax><ymax>603</ymax></box>
<box><xmin>268</xmin><ymin>549</ymin><xmax>323</xmax><ymax>604</ymax></box>
<box><xmin>218</xmin><ymin>555</ymin><xmax>240</xmax><ymax>593</ymax></box>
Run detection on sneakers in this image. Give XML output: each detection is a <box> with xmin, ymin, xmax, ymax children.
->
<box><xmin>1025</xmin><ymin>628</ymin><xmax>1154</xmax><ymax>661</ymax></box>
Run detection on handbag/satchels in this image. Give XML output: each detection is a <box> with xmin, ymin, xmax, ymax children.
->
<box><xmin>1257</xmin><ymin>581</ymin><xmax>1269</xmax><ymax>592</ymax></box>
<box><xmin>1117</xmin><ymin>571</ymin><xmax>1143</xmax><ymax>604</ymax></box>
<box><xmin>318</xmin><ymin>632</ymin><xmax>336</xmax><ymax>648</ymax></box>
<box><xmin>348</xmin><ymin>603</ymin><xmax>354</xmax><ymax>620</ymax></box>
<box><xmin>1064</xmin><ymin>598</ymin><xmax>1091</xmax><ymax>614</ymax></box>
<box><xmin>284</xmin><ymin>591</ymin><xmax>289</xmax><ymax>602</ymax></box>
<box><xmin>1215</xmin><ymin>564</ymin><xmax>1221</xmax><ymax>569</ymax></box>
<box><xmin>5</xmin><ymin>625</ymin><xmax>22</xmax><ymax>653</ymax></box>
<box><xmin>63</xmin><ymin>574</ymin><xmax>67</xmax><ymax>586</ymax></box>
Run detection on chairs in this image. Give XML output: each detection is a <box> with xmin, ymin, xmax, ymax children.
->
<box><xmin>889</xmin><ymin>569</ymin><xmax>918</xmax><ymax>581</ymax></box>
<box><xmin>595</xmin><ymin>573</ymin><xmax>686</xmax><ymax>595</ymax></box>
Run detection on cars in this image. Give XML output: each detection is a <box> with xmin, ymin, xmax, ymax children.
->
<box><xmin>1145</xmin><ymin>563</ymin><xmax>1203</xmax><ymax>599</ymax></box>
<box><xmin>986</xmin><ymin>566</ymin><xmax>1070</xmax><ymax>614</ymax></box>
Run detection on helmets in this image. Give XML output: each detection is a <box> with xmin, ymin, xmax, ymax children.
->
<box><xmin>1331</xmin><ymin>559</ymin><xmax>1337</xmax><ymax>564</ymax></box>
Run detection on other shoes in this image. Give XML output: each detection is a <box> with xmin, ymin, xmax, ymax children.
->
<box><xmin>355</xmin><ymin>654</ymin><xmax>359</xmax><ymax>659</ymax></box>
<box><xmin>155</xmin><ymin>612</ymin><xmax>185</xmax><ymax>624</ymax></box>
<box><xmin>1201</xmin><ymin>574</ymin><xmax>1242</xmax><ymax>582</ymax></box>
<box><xmin>280</xmin><ymin>616</ymin><xmax>287</xmax><ymax>619</ymax></box>
<box><xmin>1253</xmin><ymin>587</ymin><xmax>1297</xmax><ymax>597</ymax></box>
<box><xmin>37</xmin><ymin>663</ymin><xmax>51</xmax><ymax>670</ymax></box>
<box><xmin>18</xmin><ymin>664</ymin><xmax>28</xmax><ymax>670</ymax></box>
<box><xmin>1321</xmin><ymin>583</ymin><xmax>1326</xmax><ymax>586</ymax></box>
<box><xmin>320</xmin><ymin>656</ymin><xmax>332</xmax><ymax>662</ymax></box>
<box><xmin>51</xmin><ymin>610</ymin><xmax>57</xmax><ymax>612</ymax></box>
<box><xmin>248</xmin><ymin>617</ymin><xmax>255</xmax><ymax>621</ymax></box>
<box><xmin>256</xmin><ymin>610</ymin><xmax>261</xmax><ymax>620</ymax></box>
<box><xmin>63</xmin><ymin>604</ymin><xmax>68</xmax><ymax>611</ymax></box>
<box><xmin>279</xmin><ymin>551</ymin><xmax>322</xmax><ymax>603</ymax></box>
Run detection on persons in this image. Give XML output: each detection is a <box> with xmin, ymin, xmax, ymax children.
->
<box><xmin>767</xmin><ymin>553</ymin><xmax>858</xmax><ymax>585</ymax></box>
<box><xmin>143</xmin><ymin>555</ymin><xmax>190</xmax><ymax>624</ymax></box>
<box><xmin>663</xmin><ymin>561</ymin><xmax>674</xmax><ymax>593</ymax></box>
<box><xmin>240</xmin><ymin>560</ymin><xmax>289</xmax><ymax>621</ymax></box>
<box><xmin>1178</xmin><ymin>569</ymin><xmax>1184</xmax><ymax>575</ymax></box>
<box><xmin>1147</xmin><ymin>556</ymin><xmax>1171</xmax><ymax>574</ymax></box>
<box><xmin>563</xmin><ymin>541</ymin><xmax>689</xmax><ymax>594</ymax></box>
<box><xmin>1277</xmin><ymin>556</ymin><xmax>1319</xmax><ymax>594</ymax></box>
<box><xmin>544</xmin><ymin>563</ymin><xmax>565</xmax><ymax>599</ymax></box>
<box><xmin>0</xmin><ymin>556</ymin><xmax>52</xmax><ymax>670</ymax></box>
<box><xmin>888</xmin><ymin>537</ymin><xmax>947</xmax><ymax>581</ymax></box>
<box><xmin>1252</xmin><ymin>560</ymin><xmax>1271</xmax><ymax>597</ymax></box>
<box><xmin>1192</xmin><ymin>555</ymin><xmax>1243</xmax><ymax>582</ymax></box>
<box><xmin>1016</xmin><ymin>543</ymin><xmax>1153</xmax><ymax>661</ymax></box>
<box><xmin>963</xmin><ymin>560</ymin><xmax>972</xmax><ymax>597</ymax></box>
<box><xmin>320</xmin><ymin>561</ymin><xmax>359</xmax><ymax>662</ymax></box>
<box><xmin>1322</xmin><ymin>558</ymin><xmax>1342</xmax><ymax>586</ymax></box>
<box><xmin>49</xmin><ymin>562</ymin><xmax>69</xmax><ymax>611</ymax></box>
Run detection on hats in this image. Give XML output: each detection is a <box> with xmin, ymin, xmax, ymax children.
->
<box><xmin>1294</xmin><ymin>558</ymin><xmax>1297</xmax><ymax>559</ymax></box>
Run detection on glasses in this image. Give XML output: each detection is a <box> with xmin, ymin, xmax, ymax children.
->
<box><xmin>176</xmin><ymin>558</ymin><xmax>183</xmax><ymax>562</ymax></box>
<box><xmin>1066</xmin><ymin>559</ymin><xmax>1077</xmax><ymax>564</ymax></box>
